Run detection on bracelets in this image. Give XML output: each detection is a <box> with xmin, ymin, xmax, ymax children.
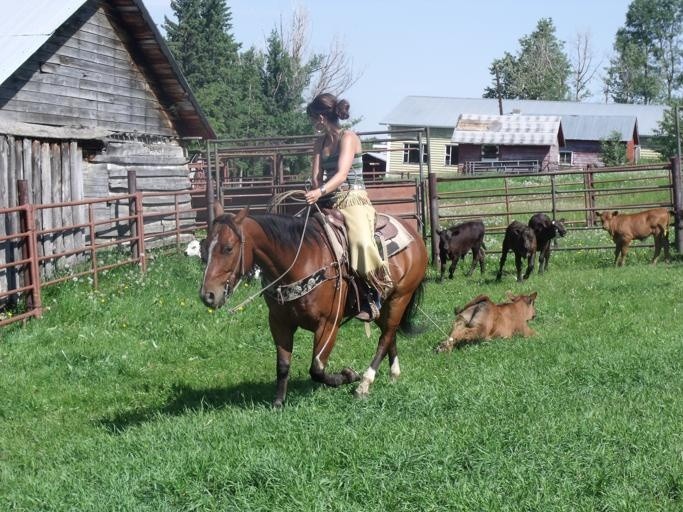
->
<box><xmin>320</xmin><ymin>185</ymin><xmax>326</xmax><ymax>197</ymax></box>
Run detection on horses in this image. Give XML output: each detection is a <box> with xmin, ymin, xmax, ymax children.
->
<box><xmin>195</xmin><ymin>198</ymin><xmax>431</xmax><ymax>407</ymax></box>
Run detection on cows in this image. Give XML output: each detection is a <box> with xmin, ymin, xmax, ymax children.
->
<box><xmin>184</xmin><ymin>238</ymin><xmax>203</xmax><ymax>260</ymax></box>
<box><xmin>432</xmin><ymin>287</ymin><xmax>540</xmax><ymax>354</ymax></box>
<box><xmin>495</xmin><ymin>212</ymin><xmax>568</xmax><ymax>283</ymax></box>
<box><xmin>435</xmin><ymin>218</ymin><xmax>487</xmax><ymax>282</ymax></box>
<box><xmin>594</xmin><ymin>206</ymin><xmax>679</xmax><ymax>266</ymax></box>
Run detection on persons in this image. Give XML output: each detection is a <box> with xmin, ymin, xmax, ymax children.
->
<box><xmin>304</xmin><ymin>93</ymin><xmax>394</xmax><ymax>321</ymax></box>
